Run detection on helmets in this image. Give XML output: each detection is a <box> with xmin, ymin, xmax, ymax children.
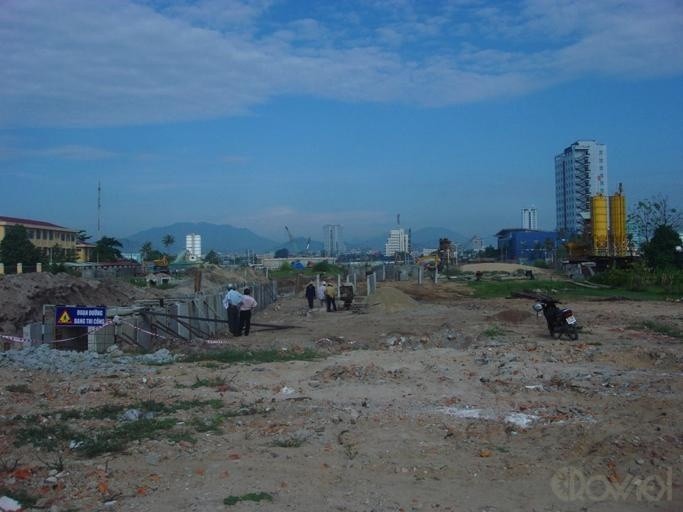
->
<box><xmin>227</xmin><ymin>283</ymin><xmax>236</xmax><ymax>291</ymax></box>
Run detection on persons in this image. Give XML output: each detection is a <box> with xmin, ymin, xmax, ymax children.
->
<box><xmin>324</xmin><ymin>282</ymin><xmax>337</xmax><ymax>312</ymax></box>
<box><xmin>316</xmin><ymin>280</ymin><xmax>326</xmax><ymax>309</ymax></box>
<box><xmin>305</xmin><ymin>282</ymin><xmax>315</xmax><ymax>310</ymax></box>
<box><xmin>222</xmin><ymin>282</ymin><xmax>242</xmax><ymax>332</ymax></box>
<box><xmin>234</xmin><ymin>288</ymin><xmax>258</xmax><ymax>337</ymax></box>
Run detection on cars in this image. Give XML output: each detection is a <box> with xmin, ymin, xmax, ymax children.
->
<box><xmin>447</xmin><ymin>267</ymin><xmax>538</xmax><ymax>284</ymax></box>
<box><xmin>170</xmin><ymin>266</ymin><xmax>185</xmax><ymax>275</ymax></box>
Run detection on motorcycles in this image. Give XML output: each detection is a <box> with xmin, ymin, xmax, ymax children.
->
<box><xmin>532</xmin><ymin>294</ymin><xmax>582</xmax><ymax>341</ymax></box>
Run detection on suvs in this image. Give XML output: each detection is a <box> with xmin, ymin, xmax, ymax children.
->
<box><xmin>147</xmin><ymin>264</ymin><xmax>169</xmax><ymax>276</ymax></box>
